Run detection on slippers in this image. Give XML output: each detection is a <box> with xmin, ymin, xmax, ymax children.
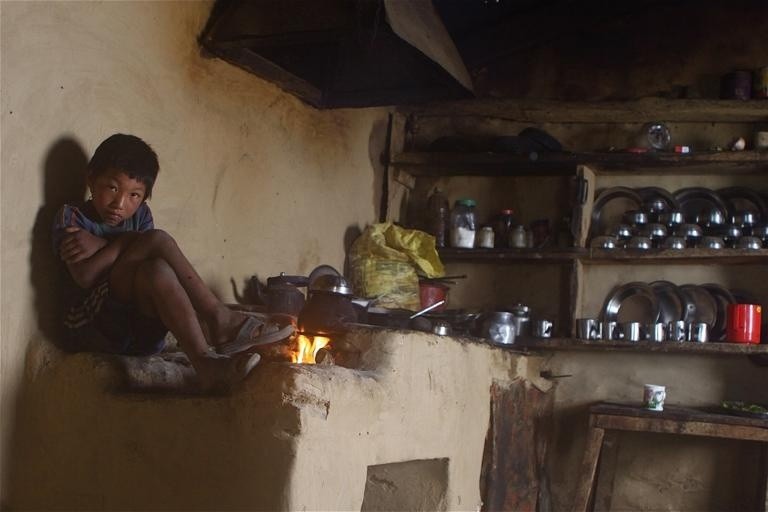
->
<box><xmin>212</xmin><ymin>315</ymin><xmax>296</xmax><ymax>357</ymax></box>
<box><xmin>198</xmin><ymin>348</ymin><xmax>262</xmax><ymax>393</ymax></box>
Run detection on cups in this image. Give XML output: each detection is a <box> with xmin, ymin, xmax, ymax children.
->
<box><xmin>536</xmin><ymin>318</ymin><xmax>553</xmax><ymax>337</ymax></box>
<box><xmin>728</xmin><ymin>304</ymin><xmax>762</xmax><ymax>344</ymax></box>
<box><xmin>752</xmin><ymin>132</ymin><xmax>768</xmax><ymax>149</ymax></box>
<box><xmin>577</xmin><ymin>318</ymin><xmax>597</xmax><ymax>342</ymax></box>
<box><xmin>641</xmin><ymin>385</ymin><xmax>665</xmax><ymax>414</ymax></box>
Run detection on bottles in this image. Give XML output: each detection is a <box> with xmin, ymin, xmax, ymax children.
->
<box><xmin>482</xmin><ymin>302</ymin><xmax>531</xmax><ymax>343</ymax></box>
<box><xmin>427</xmin><ymin>187</ymin><xmax>528</xmax><ymax>247</ymax></box>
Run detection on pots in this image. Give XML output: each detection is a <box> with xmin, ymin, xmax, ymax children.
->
<box><xmin>420</xmin><ymin>283</ymin><xmax>449</xmax><ymax>312</ymax></box>
<box><xmin>308</xmin><ymin>289</ymin><xmax>418</xmax><ymax>333</ymax></box>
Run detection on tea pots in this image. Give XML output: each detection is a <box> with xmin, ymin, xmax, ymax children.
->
<box><xmin>250</xmin><ymin>271</ymin><xmax>304</xmax><ymax>312</ymax></box>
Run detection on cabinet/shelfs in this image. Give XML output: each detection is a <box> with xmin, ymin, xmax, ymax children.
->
<box><xmin>382</xmin><ymin>110</ymin><xmax>768</xmax><ymax>356</ymax></box>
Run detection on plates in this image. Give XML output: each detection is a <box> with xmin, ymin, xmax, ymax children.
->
<box><xmin>722</xmin><ymin>406</ymin><xmax>767</xmax><ymax>419</ymax></box>
<box><xmin>588</xmin><ymin>186</ymin><xmax>768</xmax><ymax>249</ymax></box>
<box><xmin>602</xmin><ymin>281</ymin><xmax>755</xmax><ymax>341</ymax></box>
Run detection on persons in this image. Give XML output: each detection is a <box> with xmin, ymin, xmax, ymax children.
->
<box><xmin>39</xmin><ymin>133</ymin><xmax>296</xmax><ymax>391</ymax></box>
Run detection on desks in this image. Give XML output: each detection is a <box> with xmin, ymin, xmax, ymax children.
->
<box><xmin>567</xmin><ymin>396</ymin><xmax>768</xmax><ymax>512</ymax></box>
